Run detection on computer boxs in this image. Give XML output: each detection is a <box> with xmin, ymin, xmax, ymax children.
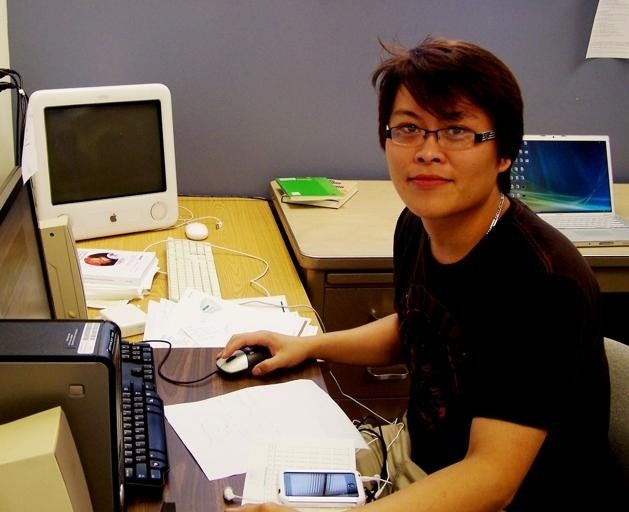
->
<box><xmin>0</xmin><ymin>318</ymin><xmax>124</xmax><ymax>512</ymax></box>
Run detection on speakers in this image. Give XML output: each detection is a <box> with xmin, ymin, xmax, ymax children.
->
<box><xmin>38</xmin><ymin>215</ymin><xmax>88</xmax><ymax>319</ymax></box>
<box><xmin>1</xmin><ymin>405</ymin><xmax>94</xmax><ymax>512</ymax></box>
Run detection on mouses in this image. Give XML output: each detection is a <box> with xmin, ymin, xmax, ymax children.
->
<box><xmin>216</xmin><ymin>343</ymin><xmax>271</xmax><ymax>378</ymax></box>
<box><xmin>185</xmin><ymin>222</ymin><xmax>209</xmax><ymax>240</ymax></box>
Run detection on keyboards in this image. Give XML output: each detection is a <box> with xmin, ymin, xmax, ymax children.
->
<box><xmin>120</xmin><ymin>339</ymin><xmax>168</xmax><ymax>492</ymax></box>
<box><xmin>166</xmin><ymin>238</ymin><xmax>222</xmax><ymax>301</ymax></box>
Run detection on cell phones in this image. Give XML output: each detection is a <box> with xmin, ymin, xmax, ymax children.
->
<box><xmin>277</xmin><ymin>470</ymin><xmax>366</xmax><ymax>506</ymax></box>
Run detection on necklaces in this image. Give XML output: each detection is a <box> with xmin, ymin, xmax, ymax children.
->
<box><xmin>489</xmin><ymin>191</ymin><xmax>507</xmax><ymax>234</ymax></box>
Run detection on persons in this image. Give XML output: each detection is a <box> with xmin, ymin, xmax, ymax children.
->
<box><xmin>213</xmin><ymin>34</ymin><xmax>614</xmax><ymax>512</ymax></box>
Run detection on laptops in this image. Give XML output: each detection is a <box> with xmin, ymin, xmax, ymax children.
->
<box><xmin>508</xmin><ymin>134</ymin><xmax>629</xmax><ymax>248</ymax></box>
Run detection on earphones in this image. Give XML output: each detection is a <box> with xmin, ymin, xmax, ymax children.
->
<box><xmin>224</xmin><ymin>487</ymin><xmax>234</xmax><ymax>501</ymax></box>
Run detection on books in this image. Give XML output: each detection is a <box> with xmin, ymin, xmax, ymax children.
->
<box><xmin>75</xmin><ymin>246</ymin><xmax>159</xmax><ymax>309</ymax></box>
<box><xmin>280</xmin><ymin>178</ymin><xmax>359</xmax><ymax>210</ymax></box>
<box><xmin>274</xmin><ymin>175</ymin><xmax>344</xmax><ymax>201</ymax></box>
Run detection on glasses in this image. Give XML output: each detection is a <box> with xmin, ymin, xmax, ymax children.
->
<box><xmin>384</xmin><ymin>123</ymin><xmax>495</xmax><ymax>152</ymax></box>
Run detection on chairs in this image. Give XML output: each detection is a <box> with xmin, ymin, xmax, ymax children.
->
<box><xmin>602</xmin><ymin>335</ymin><xmax>629</xmax><ymax>470</ymax></box>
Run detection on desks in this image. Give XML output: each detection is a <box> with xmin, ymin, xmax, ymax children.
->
<box><xmin>268</xmin><ymin>176</ymin><xmax>629</xmax><ymax>427</ymax></box>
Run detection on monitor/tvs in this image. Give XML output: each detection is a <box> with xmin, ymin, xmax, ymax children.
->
<box><xmin>0</xmin><ymin>166</ymin><xmax>56</xmax><ymax>320</ymax></box>
<box><xmin>22</xmin><ymin>82</ymin><xmax>179</xmax><ymax>241</ymax></box>
